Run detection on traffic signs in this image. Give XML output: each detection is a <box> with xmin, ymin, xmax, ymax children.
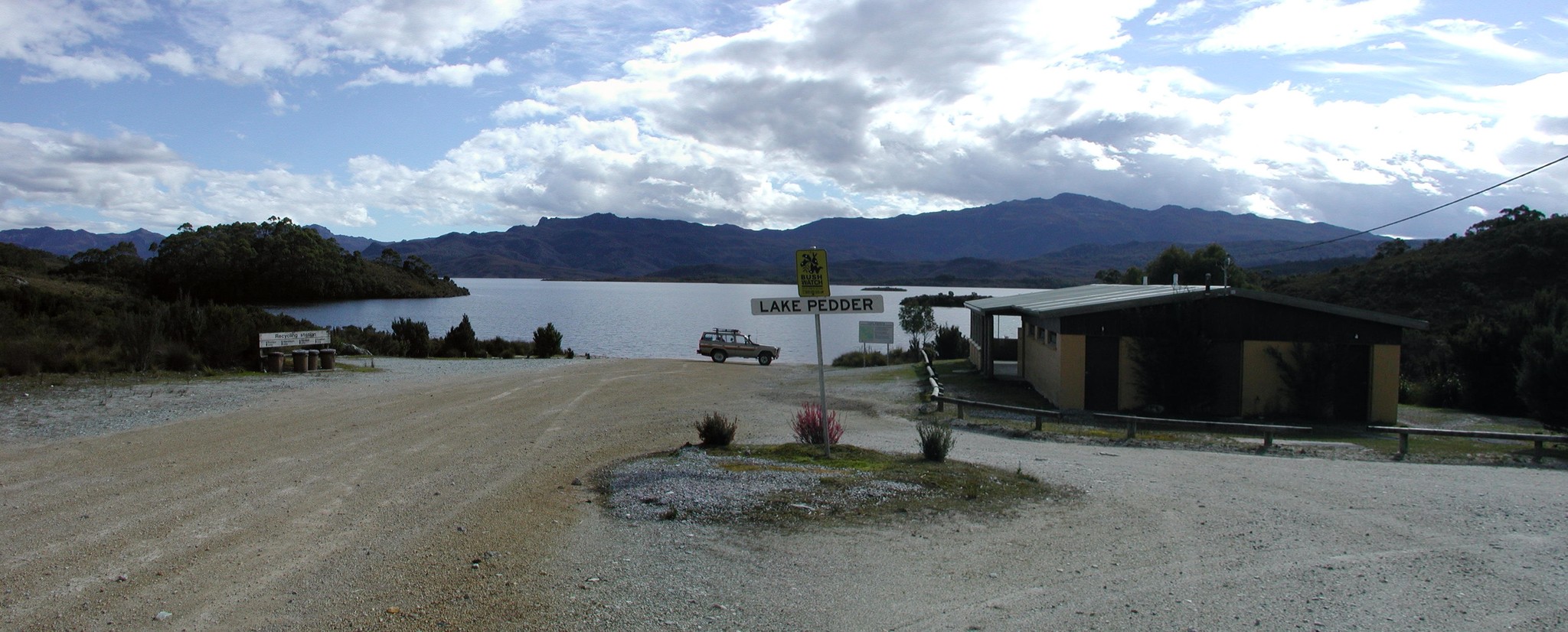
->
<box><xmin>750</xmin><ymin>294</ymin><xmax>885</xmax><ymax>315</ymax></box>
<box><xmin>795</xmin><ymin>248</ymin><xmax>832</xmax><ymax>297</ymax></box>
<box><xmin>258</xmin><ymin>330</ymin><xmax>331</xmax><ymax>348</ymax></box>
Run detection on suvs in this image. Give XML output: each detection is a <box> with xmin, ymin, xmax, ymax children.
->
<box><xmin>696</xmin><ymin>328</ymin><xmax>781</xmax><ymax>365</ymax></box>
